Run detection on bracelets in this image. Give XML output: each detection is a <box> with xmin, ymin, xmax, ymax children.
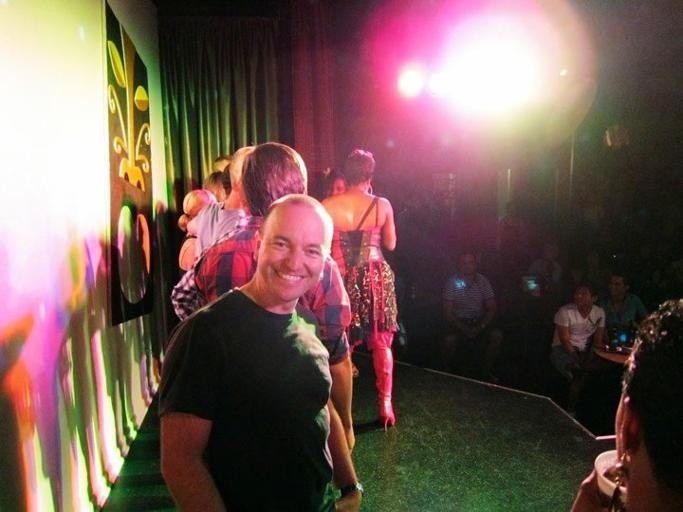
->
<box><xmin>337</xmin><ymin>482</ymin><xmax>364</xmax><ymax>496</ymax></box>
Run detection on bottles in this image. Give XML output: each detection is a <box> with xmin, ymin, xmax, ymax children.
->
<box><xmin>610</xmin><ymin>323</ymin><xmax>636</xmax><ymax>354</ymax></box>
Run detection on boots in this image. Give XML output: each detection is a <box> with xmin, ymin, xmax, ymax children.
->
<box><xmin>371</xmin><ymin>346</ymin><xmax>396</xmax><ymax>433</ymax></box>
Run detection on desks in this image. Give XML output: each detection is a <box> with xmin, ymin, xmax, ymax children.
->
<box><xmin>593</xmin><ymin>344</ymin><xmax>631</xmax><ymax>429</ymax></box>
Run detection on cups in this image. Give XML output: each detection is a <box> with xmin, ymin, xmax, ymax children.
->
<box><xmin>593</xmin><ymin>446</ymin><xmax>627</xmax><ymax>507</ymax></box>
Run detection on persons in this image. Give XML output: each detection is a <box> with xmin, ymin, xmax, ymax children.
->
<box><xmin>156</xmin><ymin>195</ymin><xmax>364</xmax><ymax>512</ymax></box>
<box><xmin>171</xmin><ymin>140</ymin><xmax>358</xmax><ymax>457</ymax></box>
<box><xmin>320</xmin><ymin>149</ymin><xmax>397</xmax><ymax>431</ymax></box>
<box><xmin>569</xmin><ymin>298</ymin><xmax>683</xmax><ymax>512</ymax></box>
<box><xmin>395</xmin><ymin>196</ymin><xmax>683</xmax><ymax>430</ymax></box>
